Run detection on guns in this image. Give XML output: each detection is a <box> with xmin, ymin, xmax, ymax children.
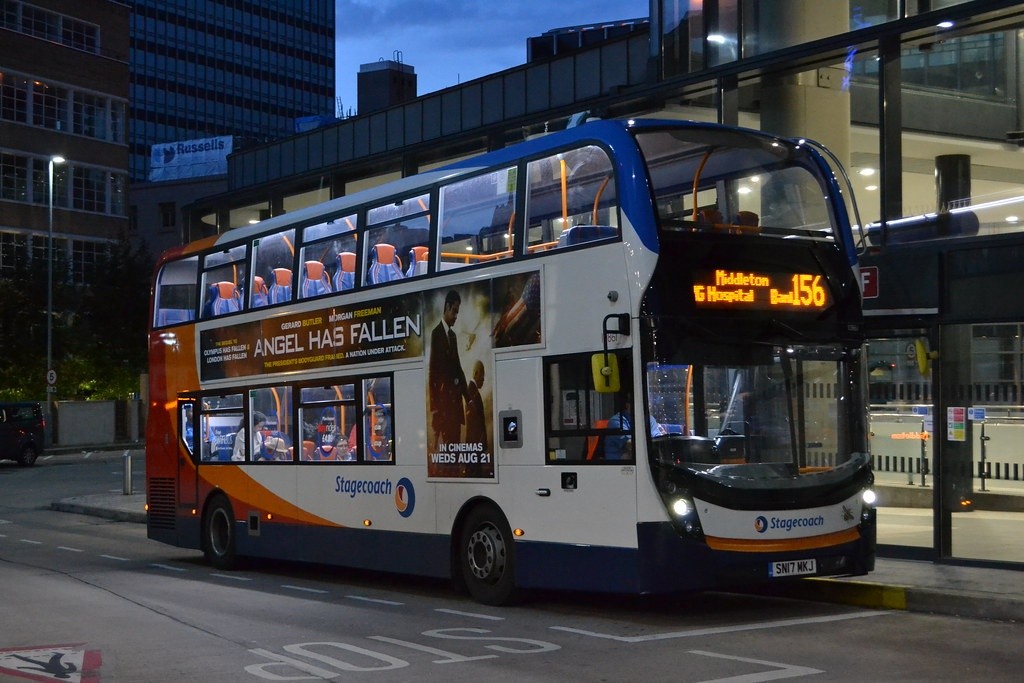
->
<box><xmin>463</xmin><ymin>430</ymin><xmax>487</xmax><ymax>477</ymax></box>
<box><xmin>433</xmin><ymin>382</ymin><xmax>447</xmax><ymax>450</ymax></box>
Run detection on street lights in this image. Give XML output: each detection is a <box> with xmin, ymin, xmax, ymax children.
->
<box><xmin>47</xmin><ymin>156</ymin><xmax>67</xmax><ymax>449</ymax></box>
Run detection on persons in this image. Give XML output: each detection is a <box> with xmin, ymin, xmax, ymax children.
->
<box><xmin>256</xmin><ymin>436</ymin><xmax>288</xmax><ymax>461</ymax></box>
<box><xmin>429</xmin><ymin>289</ymin><xmax>473</xmax><ymax>478</ymax></box>
<box><xmin>462</xmin><ymin>358</ymin><xmax>488</xmax><ymax>477</ymax></box>
<box><xmin>184</xmin><ymin>403</ymin><xmax>215</xmax><ymax>452</ymax></box>
<box><xmin>333</xmin><ymin>435</ymin><xmax>352</xmax><ymax>461</ymax></box>
<box><xmin>230</xmin><ymin>410</ymin><xmax>267</xmax><ymax>461</ymax></box>
<box><xmin>604</xmin><ymin>391</ymin><xmax>663</xmax><ymax>460</ymax></box>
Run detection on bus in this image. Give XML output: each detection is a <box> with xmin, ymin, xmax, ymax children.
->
<box><xmin>145</xmin><ymin>116</ymin><xmax>940</xmax><ymax>613</ymax></box>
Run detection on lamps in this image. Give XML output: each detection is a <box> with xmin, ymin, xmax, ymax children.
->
<box><xmin>706</xmin><ymin>29</ymin><xmax>741</xmax><ymax>49</ymax></box>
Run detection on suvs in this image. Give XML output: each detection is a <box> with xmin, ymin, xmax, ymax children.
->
<box><xmin>0</xmin><ymin>402</ymin><xmax>45</xmax><ymax>467</ymax></box>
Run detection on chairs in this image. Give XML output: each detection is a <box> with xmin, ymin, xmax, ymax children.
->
<box><xmin>680</xmin><ymin>206</ymin><xmax>759</xmax><ymax>236</ymax></box>
<box><xmin>212</xmin><ymin>425</ymin><xmax>388</xmax><ymax>462</ymax></box>
<box><xmin>558</xmin><ymin>225</ymin><xmax>620</xmax><ymax>246</ymax></box>
<box><xmin>156</xmin><ymin>244</ymin><xmax>426</xmax><ymax>326</ymax></box>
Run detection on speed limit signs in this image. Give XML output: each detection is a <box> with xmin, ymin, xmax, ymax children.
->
<box><xmin>47</xmin><ymin>370</ymin><xmax>57</xmax><ymax>385</ymax></box>
